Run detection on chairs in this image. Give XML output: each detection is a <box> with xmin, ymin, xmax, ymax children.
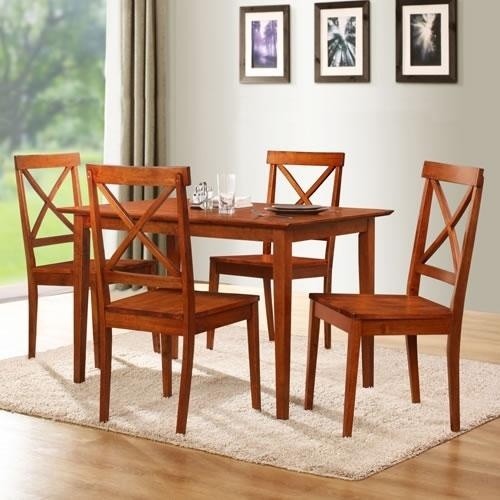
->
<box><xmin>205</xmin><ymin>148</ymin><xmax>345</xmax><ymax>350</ymax></box>
<box><xmin>303</xmin><ymin>162</ymin><xmax>485</xmax><ymax>440</ymax></box>
<box><xmin>13</xmin><ymin>150</ymin><xmax>162</xmax><ymax>371</ymax></box>
<box><xmin>79</xmin><ymin>163</ymin><xmax>264</xmax><ymax>439</ymax></box>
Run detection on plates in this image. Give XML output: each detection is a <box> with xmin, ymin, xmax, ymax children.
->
<box><xmin>265</xmin><ymin>206</ymin><xmax>329</xmax><ymax>214</ymax></box>
<box><xmin>273</xmin><ymin>204</ymin><xmax>322</xmax><ymax>210</ymax></box>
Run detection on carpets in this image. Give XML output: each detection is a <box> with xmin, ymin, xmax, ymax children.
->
<box><xmin>0</xmin><ymin>321</ymin><xmax>500</xmax><ymax>482</ymax></box>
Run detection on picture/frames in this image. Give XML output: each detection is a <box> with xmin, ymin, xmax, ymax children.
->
<box><xmin>393</xmin><ymin>0</ymin><xmax>459</xmax><ymax>86</ymax></box>
<box><xmin>238</xmin><ymin>3</ymin><xmax>291</xmax><ymax>86</ymax></box>
<box><xmin>313</xmin><ymin>1</ymin><xmax>372</xmax><ymax>84</ymax></box>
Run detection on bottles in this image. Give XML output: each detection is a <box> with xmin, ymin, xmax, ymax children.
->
<box><xmin>190</xmin><ymin>181</ymin><xmax>216</xmax><ymax>209</ymax></box>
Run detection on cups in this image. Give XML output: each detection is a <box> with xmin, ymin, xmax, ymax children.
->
<box><xmin>216</xmin><ymin>173</ymin><xmax>237</xmax><ymax>215</ymax></box>
